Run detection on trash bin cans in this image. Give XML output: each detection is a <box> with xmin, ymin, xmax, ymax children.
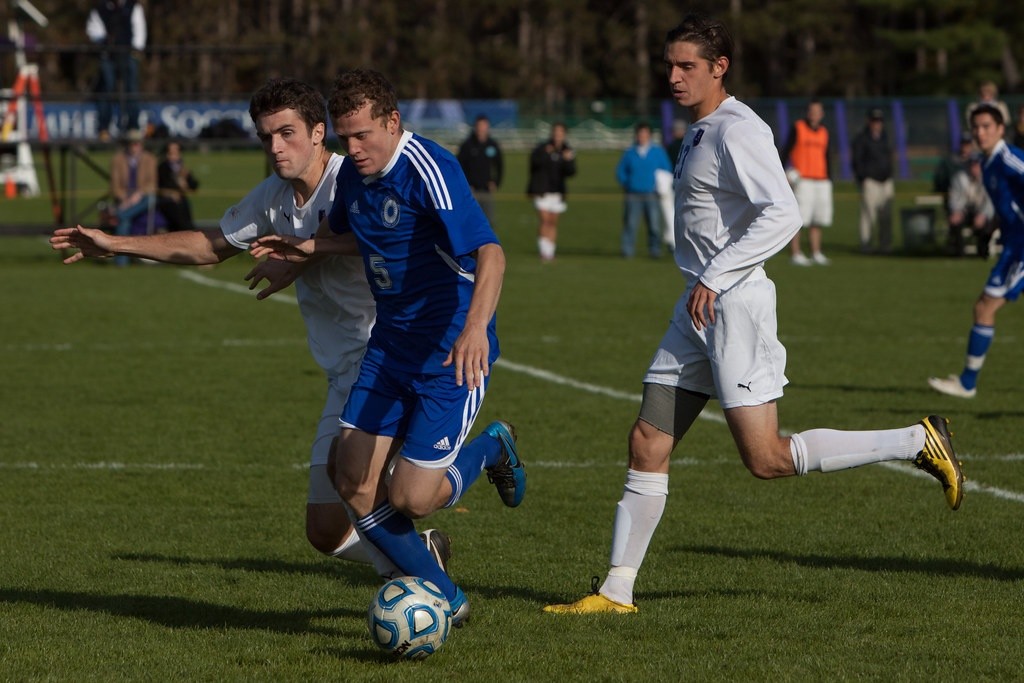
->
<box><xmin>899</xmin><ymin>206</ymin><xmax>939</xmax><ymax>258</ymax></box>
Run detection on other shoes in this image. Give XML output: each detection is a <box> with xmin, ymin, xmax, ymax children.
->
<box><xmin>537</xmin><ymin>236</ymin><xmax>555</xmax><ymax>260</ymax></box>
<box><xmin>929</xmin><ymin>376</ymin><xmax>978</xmax><ymax>399</ymax></box>
<box><xmin>790</xmin><ymin>253</ymin><xmax>811</xmax><ymax>267</ymax></box>
<box><xmin>811</xmin><ymin>254</ymin><xmax>827</xmax><ymax>264</ymax></box>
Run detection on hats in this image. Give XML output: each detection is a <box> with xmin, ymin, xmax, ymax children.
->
<box><xmin>124</xmin><ymin>129</ymin><xmax>146</xmax><ymax>145</ymax></box>
<box><xmin>868</xmin><ymin>109</ymin><xmax>883</xmax><ymax>120</ymax></box>
<box><xmin>965</xmin><ymin>98</ymin><xmax>1011</xmax><ymax>129</ymax></box>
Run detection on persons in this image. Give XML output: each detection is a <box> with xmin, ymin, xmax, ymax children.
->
<box><xmin>157</xmin><ymin>140</ymin><xmax>194</xmax><ymax>233</ymax></box>
<box><xmin>783</xmin><ymin>100</ymin><xmax>833</xmax><ymax>267</ymax></box>
<box><xmin>615</xmin><ymin>124</ymin><xmax>672</xmax><ymax>259</ymax></box>
<box><xmin>86</xmin><ymin>0</ymin><xmax>147</xmax><ymax>142</ymax></box>
<box><xmin>852</xmin><ymin>115</ymin><xmax>896</xmax><ymax>254</ymax></box>
<box><xmin>666</xmin><ymin>119</ymin><xmax>689</xmax><ymax>169</ymax></box>
<box><xmin>527</xmin><ymin>123</ymin><xmax>577</xmax><ymax>260</ymax></box>
<box><xmin>49</xmin><ymin>78</ymin><xmax>454</xmax><ymax>582</ymax></box>
<box><xmin>542</xmin><ymin>17</ymin><xmax>967</xmax><ymax>615</ymax></box>
<box><xmin>926</xmin><ymin>104</ymin><xmax>1024</xmax><ymax>398</ymax></box>
<box><xmin>940</xmin><ymin>139</ymin><xmax>998</xmax><ymax>259</ymax></box>
<box><xmin>249</xmin><ymin>65</ymin><xmax>527</xmax><ymax>628</ymax></box>
<box><xmin>111</xmin><ymin>129</ymin><xmax>157</xmax><ymax>264</ymax></box>
<box><xmin>965</xmin><ymin>81</ymin><xmax>1012</xmax><ymax>140</ymax></box>
<box><xmin>456</xmin><ymin>115</ymin><xmax>503</xmax><ymax>227</ymax></box>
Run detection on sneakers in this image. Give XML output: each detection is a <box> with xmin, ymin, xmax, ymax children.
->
<box><xmin>481</xmin><ymin>420</ymin><xmax>527</xmax><ymax>509</ymax></box>
<box><xmin>542</xmin><ymin>590</ymin><xmax>639</xmax><ymax>616</ymax></box>
<box><xmin>417</xmin><ymin>528</ymin><xmax>452</xmax><ymax>576</ymax></box>
<box><xmin>444</xmin><ymin>585</ymin><xmax>471</xmax><ymax>625</ymax></box>
<box><xmin>913</xmin><ymin>415</ymin><xmax>966</xmax><ymax>511</ymax></box>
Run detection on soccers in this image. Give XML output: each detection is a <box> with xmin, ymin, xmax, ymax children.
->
<box><xmin>368</xmin><ymin>576</ymin><xmax>453</xmax><ymax>660</ymax></box>
